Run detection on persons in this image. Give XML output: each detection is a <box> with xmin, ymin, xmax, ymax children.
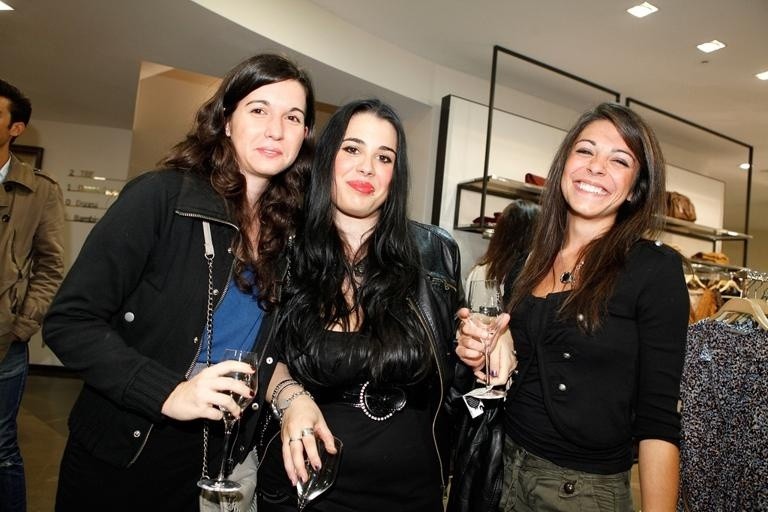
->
<box><xmin>0</xmin><ymin>79</ymin><xmax>70</xmax><ymax>511</ymax></box>
<box><xmin>41</xmin><ymin>53</ymin><xmax>317</xmax><ymax>512</ymax></box>
<box><xmin>265</xmin><ymin>98</ymin><xmax>476</xmax><ymax>512</ymax></box>
<box><xmin>455</xmin><ymin>102</ymin><xmax>690</xmax><ymax>512</ymax></box>
<box><xmin>464</xmin><ymin>201</ymin><xmax>543</xmax><ymax>391</ymax></box>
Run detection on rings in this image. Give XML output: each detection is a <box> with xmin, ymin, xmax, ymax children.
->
<box><xmin>288</xmin><ymin>437</ymin><xmax>302</xmax><ymax>446</ymax></box>
<box><xmin>301</xmin><ymin>428</ymin><xmax>314</xmax><ymax>437</ymax></box>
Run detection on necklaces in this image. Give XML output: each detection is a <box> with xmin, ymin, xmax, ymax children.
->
<box><xmin>560</xmin><ymin>253</ymin><xmax>584</xmax><ymax>284</ymax></box>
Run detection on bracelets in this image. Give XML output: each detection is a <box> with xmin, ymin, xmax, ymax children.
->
<box><xmin>279</xmin><ymin>390</ymin><xmax>314</xmax><ymax>424</ymax></box>
<box><xmin>270</xmin><ymin>378</ymin><xmax>303</xmax><ymax>417</ymax></box>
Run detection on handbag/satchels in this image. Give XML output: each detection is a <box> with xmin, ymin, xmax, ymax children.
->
<box><xmin>666</xmin><ymin>190</ymin><xmax>696</xmax><ymax>221</ymax></box>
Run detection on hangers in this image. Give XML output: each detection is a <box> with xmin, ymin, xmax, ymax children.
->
<box><xmin>686</xmin><ymin>268</ymin><xmax>768</xmax><ymax>332</ymax></box>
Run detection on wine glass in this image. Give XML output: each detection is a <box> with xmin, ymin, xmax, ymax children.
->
<box><xmin>292</xmin><ymin>430</ymin><xmax>344</xmax><ymax>512</ymax></box>
<box><xmin>464</xmin><ymin>278</ymin><xmax>508</xmax><ymax>401</ymax></box>
<box><xmin>197</xmin><ymin>346</ymin><xmax>261</xmax><ymax>494</ymax></box>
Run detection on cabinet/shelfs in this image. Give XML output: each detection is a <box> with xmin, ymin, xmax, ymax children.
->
<box><xmin>453</xmin><ymin>175</ymin><xmax>748</xmax><ymax>300</ymax></box>
